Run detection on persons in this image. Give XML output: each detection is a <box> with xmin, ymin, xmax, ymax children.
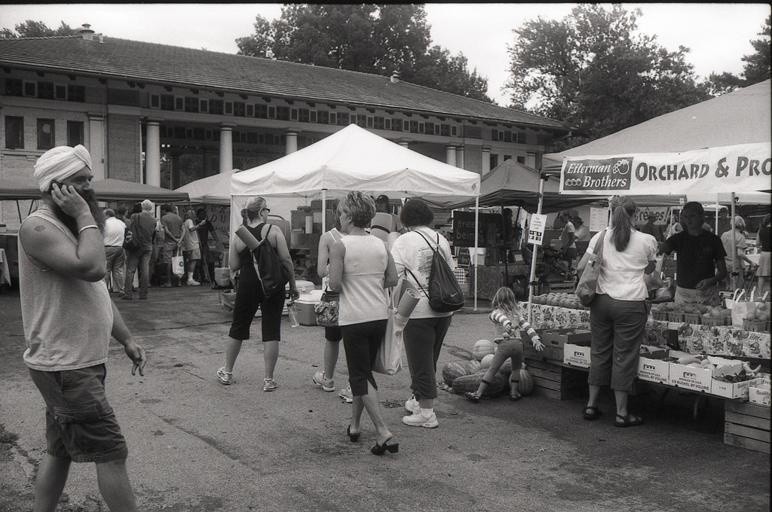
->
<box><xmin>318</xmin><ymin>191</ymin><xmax>454</xmax><ymax>458</ymax></box>
<box><xmin>500</xmin><ymin>208</ymin><xmax>514</xmax><ymax>236</ymax></box>
<box><xmin>104</xmin><ymin>200</ymin><xmax>219</xmax><ymax>301</ymax></box>
<box><xmin>18</xmin><ymin>143</ymin><xmax>148</xmax><ymax>510</ymax></box>
<box><xmin>559</xmin><ymin>195</ymin><xmax>770</xmax><ymax>426</ymax></box>
<box><xmin>217</xmin><ymin>199</ymin><xmax>300</xmax><ymax>391</ymax></box>
<box><xmin>463</xmin><ymin>285</ymin><xmax>546</xmax><ymax>404</ymax></box>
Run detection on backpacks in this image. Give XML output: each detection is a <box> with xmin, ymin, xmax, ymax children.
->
<box><xmin>405</xmin><ymin>230</ymin><xmax>465</xmax><ymax>312</ymax></box>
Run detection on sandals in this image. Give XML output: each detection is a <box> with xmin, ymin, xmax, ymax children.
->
<box><xmin>614</xmin><ymin>412</ymin><xmax>644</xmax><ymax>426</ymax></box>
<box><xmin>584</xmin><ymin>405</ymin><xmax>600</xmax><ymax>421</ymax></box>
<box><xmin>464</xmin><ymin>391</ymin><xmax>481</xmax><ymax>402</ymax></box>
<box><xmin>172</xmin><ymin>256</ymin><xmax>184</xmax><ymax>276</ymax></box>
<box><xmin>511</xmin><ymin>392</ymin><xmax>522</xmax><ymax>400</ymax></box>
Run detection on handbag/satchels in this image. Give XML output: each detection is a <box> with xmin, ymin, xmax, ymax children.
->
<box><xmin>314</xmin><ymin>300</ymin><xmax>339</xmax><ymax>327</ymax></box>
<box><xmin>371</xmin><ymin>306</ymin><xmax>409</xmax><ymax>376</ymax></box>
<box><xmin>250</xmin><ymin>237</ymin><xmax>289</xmax><ymax>298</ymax></box>
<box><xmin>574</xmin><ymin>253</ymin><xmax>603</xmax><ymax>307</ymax></box>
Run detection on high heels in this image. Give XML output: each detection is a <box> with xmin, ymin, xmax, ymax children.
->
<box><xmin>371</xmin><ymin>435</ymin><xmax>400</xmax><ymax>456</ymax></box>
<box><xmin>347</xmin><ymin>424</ymin><xmax>360</xmax><ymax>441</ymax></box>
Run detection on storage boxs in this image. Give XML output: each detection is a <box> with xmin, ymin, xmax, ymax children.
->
<box><xmin>289</xmin><ymin>294</ymin><xmax>321</xmax><ymax>327</ymax></box>
<box><xmin>523</xmin><ymin>327</ymin><xmax>772</xmax><ymax>407</ymax></box>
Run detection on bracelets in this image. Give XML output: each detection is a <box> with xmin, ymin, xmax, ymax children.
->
<box><xmin>77</xmin><ymin>223</ymin><xmax>99</xmax><ymax>236</ymax></box>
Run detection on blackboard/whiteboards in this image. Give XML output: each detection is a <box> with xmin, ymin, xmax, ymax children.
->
<box><xmin>451</xmin><ymin>210</ymin><xmax>507</xmax><ymax>248</ymax></box>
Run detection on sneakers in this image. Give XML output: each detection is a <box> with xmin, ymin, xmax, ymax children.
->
<box><xmin>313</xmin><ymin>371</ymin><xmax>336</xmax><ymax>392</ymax></box>
<box><xmin>338</xmin><ymin>384</ymin><xmax>353</xmax><ymax>403</ymax></box>
<box><xmin>402</xmin><ymin>394</ymin><xmax>439</xmax><ymax>428</ymax></box>
<box><xmin>217</xmin><ymin>366</ymin><xmax>233</xmax><ymax>384</ymax></box>
<box><xmin>263</xmin><ymin>376</ymin><xmax>278</xmax><ymax>392</ymax></box>
<box><xmin>186</xmin><ymin>279</ymin><xmax>200</xmax><ymax>286</ymax></box>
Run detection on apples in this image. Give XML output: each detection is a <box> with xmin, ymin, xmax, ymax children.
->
<box><xmin>529</xmin><ymin>293</ymin><xmax>591</xmax><ymax>311</ymax></box>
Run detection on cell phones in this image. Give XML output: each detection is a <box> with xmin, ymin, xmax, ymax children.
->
<box><xmin>47</xmin><ymin>181</ymin><xmax>79</xmax><ymax>196</ymax></box>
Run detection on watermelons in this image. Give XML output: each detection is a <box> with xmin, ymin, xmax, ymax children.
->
<box><xmin>442</xmin><ymin>339</ymin><xmax>534</xmax><ymax>398</ymax></box>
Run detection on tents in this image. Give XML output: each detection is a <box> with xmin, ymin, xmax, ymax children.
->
<box><xmin>528</xmin><ymin>81</ymin><xmax>771</xmax><ymax>329</ymax></box>
<box><xmin>455</xmin><ymin>159</ymin><xmax>600</xmax><ymax>288</ymax></box>
<box><xmin>226</xmin><ymin>123</ymin><xmax>481</xmax><ymax>311</ymax></box>
<box><xmin>91</xmin><ymin>178</ymin><xmax>213</xmax><ymax>285</ymax></box>
<box><xmin>175</xmin><ymin>168</ymin><xmax>244</xmax><ymax>205</ymax></box>
